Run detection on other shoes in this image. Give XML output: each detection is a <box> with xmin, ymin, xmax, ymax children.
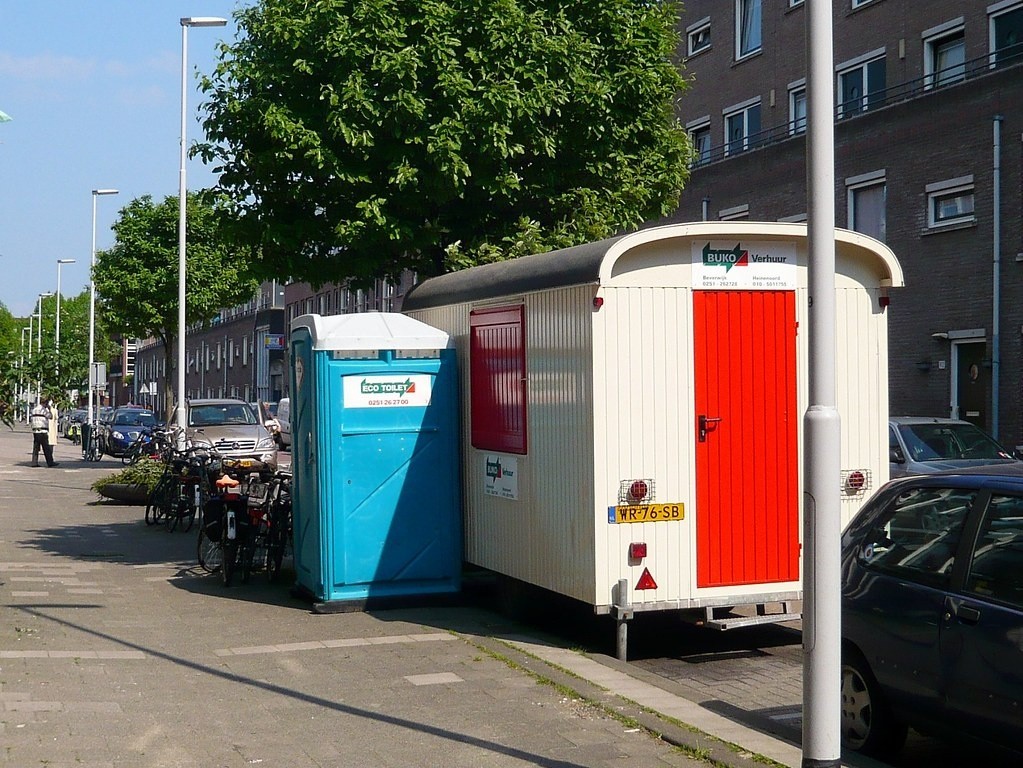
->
<box><xmin>49</xmin><ymin>462</ymin><xmax>60</xmax><ymax>467</ymax></box>
<box><xmin>32</xmin><ymin>464</ymin><xmax>41</xmax><ymax>467</ymax></box>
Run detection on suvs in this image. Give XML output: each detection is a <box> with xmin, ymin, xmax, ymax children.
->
<box><xmin>166</xmin><ymin>397</ymin><xmax>278</xmax><ymax>483</ymax></box>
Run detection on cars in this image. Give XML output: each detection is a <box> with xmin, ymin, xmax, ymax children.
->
<box><xmin>58</xmin><ymin>406</ymin><xmax>114</xmax><ymax>440</ymax></box>
<box><xmin>837</xmin><ymin>462</ymin><xmax>1023</xmax><ymax>768</ymax></box>
<box><xmin>249</xmin><ymin>402</ymin><xmax>282</xmax><ymax>442</ymax></box>
<box><xmin>104</xmin><ymin>407</ymin><xmax>158</xmax><ymax>456</ymax></box>
<box><xmin>890</xmin><ymin>416</ymin><xmax>1023</xmax><ymax>477</ymax></box>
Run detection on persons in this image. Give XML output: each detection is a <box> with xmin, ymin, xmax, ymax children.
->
<box><xmin>45</xmin><ymin>398</ymin><xmax>59</xmax><ymax>455</ymax></box>
<box><xmin>30</xmin><ymin>396</ymin><xmax>59</xmax><ymax>467</ymax></box>
<box><xmin>127</xmin><ymin>400</ymin><xmax>134</xmax><ymax>408</ymax></box>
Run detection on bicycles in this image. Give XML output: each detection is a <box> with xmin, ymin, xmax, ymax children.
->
<box><xmin>122</xmin><ymin>423</ymin><xmax>294</xmax><ymax>584</ymax></box>
<box><xmin>84</xmin><ymin>419</ymin><xmax>107</xmax><ymax>460</ymax></box>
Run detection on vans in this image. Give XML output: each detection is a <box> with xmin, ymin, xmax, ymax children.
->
<box><xmin>275</xmin><ymin>398</ymin><xmax>295</xmax><ymax>450</ymax></box>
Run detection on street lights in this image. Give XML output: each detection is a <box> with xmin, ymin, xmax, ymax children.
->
<box><xmin>54</xmin><ymin>258</ymin><xmax>77</xmax><ymax>393</ymax></box>
<box><xmin>8</xmin><ymin>351</ymin><xmax>18</xmax><ymax>421</ymax></box>
<box><xmin>86</xmin><ymin>188</ymin><xmax>120</xmax><ymax>427</ymax></box>
<box><xmin>37</xmin><ymin>292</ymin><xmax>52</xmax><ymax>407</ymax></box>
<box><xmin>26</xmin><ymin>314</ymin><xmax>41</xmax><ymax>424</ymax></box>
<box><xmin>19</xmin><ymin>327</ymin><xmax>31</xmax><ymax>423</ymax></box>
<box><xmin>177</xmin><ymin>16</ymin><xmax>232</xmax><ymax>449</ymax></box>
